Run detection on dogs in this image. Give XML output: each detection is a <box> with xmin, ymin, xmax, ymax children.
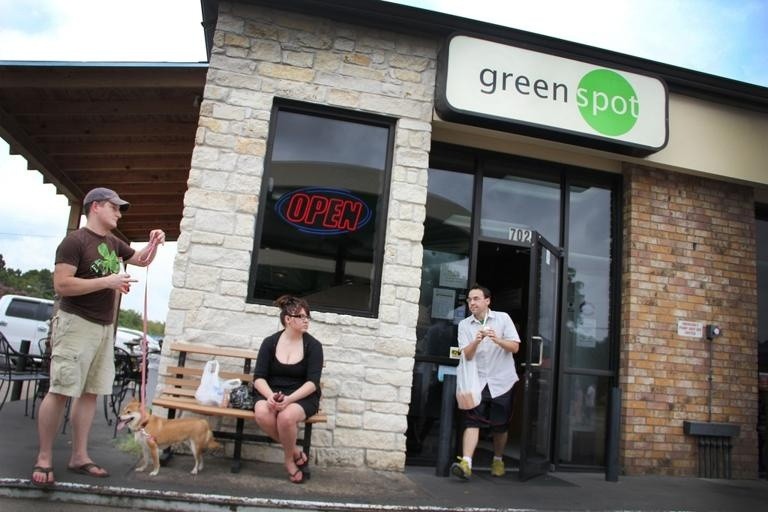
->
<box><xmin>117</xmin><ymin>394</ymin><xmax>225</xmax><ymax>475</ymax></box>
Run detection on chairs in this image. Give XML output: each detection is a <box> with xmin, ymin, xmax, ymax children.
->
<box><xmin>0</xmin><ymin>331</ymin><xmax>149</xmax><ymax>439</ymax></box>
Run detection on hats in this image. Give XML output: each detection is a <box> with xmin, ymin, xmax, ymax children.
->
<box><xmin>83</xmin><ymin>186</ymin><xmax>130</xmax><ymax>211</ymax></box>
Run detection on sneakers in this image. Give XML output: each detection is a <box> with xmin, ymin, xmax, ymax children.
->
<box><xmin>451</xmin><ymin>456</ymin><xmax>471</xmax><ymax>479</ymax></box>
<box><xmin>491</xmin><ymin>459</ymin><xmax>505</xmax><ymax>476</ymax></box>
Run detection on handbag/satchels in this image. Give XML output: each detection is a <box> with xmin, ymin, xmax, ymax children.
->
<box><xmin>230</xmin><ymin>381</ymin><xmax>267</xmax><ymax>410</ymax></box>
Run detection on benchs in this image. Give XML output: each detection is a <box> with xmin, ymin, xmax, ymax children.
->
<box><xmin>152</xmin><ymin>342</ymin><xmax>328</xmax><ymax>480</ymax></box>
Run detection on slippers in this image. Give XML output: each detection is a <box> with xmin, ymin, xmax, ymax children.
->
<box><xmin>287</xmin><ymin>449</ymin><xmax>309</xmax><ymax>484</ymax></box>
<box><xmin>68</xmin><ymin>462</ymin><xmax>110</xmax><ymax>478</ymax></box>
<box><xmin>31</xmin><ymin>461</ymin><xmax>55</xmax><ymax>487</ymax></box>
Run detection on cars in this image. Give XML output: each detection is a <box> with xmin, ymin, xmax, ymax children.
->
<box><xmin>118</xmin><ymin>325</ymin><xmax>161</xmax><ymax>355</ymax></box>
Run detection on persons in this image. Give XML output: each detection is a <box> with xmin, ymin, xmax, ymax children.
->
<box><xmin>253</xmin><ymin>297</ymin><xmax>322</xmax><ymax>484</ymax></box>
<box><xmin>31</xmin><ymin>188</ymin><xmax>165</xmax><ymax>489</ymax></box>
<box><xmin>449</xmin><ymin>286</ymin><xmax>522</xmax><ymax>481</ymax></box>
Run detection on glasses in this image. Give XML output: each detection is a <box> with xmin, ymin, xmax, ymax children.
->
<box><xmin>289</xmin><ymin>314</ymin><xmax>312</xmax><ymax>321</ymax></box>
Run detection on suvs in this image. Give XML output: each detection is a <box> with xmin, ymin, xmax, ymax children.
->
<box><xmin>0</xmin><ymin>294</ymin><xmax>145</xmax><ymax>375</ymax></box>
